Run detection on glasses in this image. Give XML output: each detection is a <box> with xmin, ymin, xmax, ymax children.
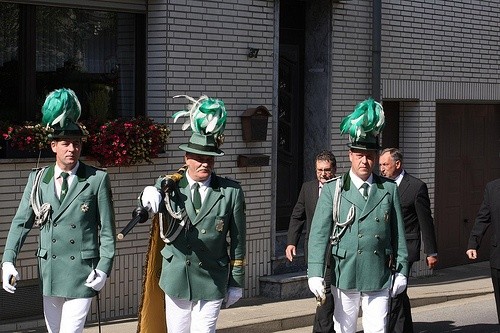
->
<box><xmin>316</xmin><ymin>168</ymin><xmax>333</xmax><ymax>173</ymax></box>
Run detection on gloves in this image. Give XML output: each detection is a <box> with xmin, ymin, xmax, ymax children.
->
<box><xmin>224</xmin><ymin>287</ymin><xmax>242</xmax><ymax>308</ymax></box>
<box><xmin>84</xmin><ymin>269</ymin><xmax>107</xmax><ymax>291</ymax></box>
<box><xmin>309</xmin><ymin>277</ymin><xmax>327</xmax><ymax>299</ymax></box>
<box><xmin>3</xmin><ymin>262</ymin><xmax>20</xmax><ymax>294</ymax></box>
<box><xmin>142</xmin><ymin>186</ymin><xmax>162</xmax><ymax>213</ymax></box>
<box><xmin>391</xmin><ymin>273</ymin><xmax>407</xmax><ymax>297</ymax></box>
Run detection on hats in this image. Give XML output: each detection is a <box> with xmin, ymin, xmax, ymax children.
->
<box><xmin>172</xmin><ymin>95</ymin><xmax>227</xmax><ymax>156</ymax></box>
<box><xmin>41</xmin><ymin>88</ymin><xmax>85</xmax><ymax>138</ymax></box>
<box><xmin>340</xmin><ymin>97</ymin><xmax>385</xmax><ymax>150</ymax></box>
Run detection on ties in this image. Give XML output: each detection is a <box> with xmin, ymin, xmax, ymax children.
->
<box><xmin>60</xmin><ymin>172</ymin><xmax>69</xmax><ymax>205</ymax></box>
<box><xmin>193</xmin><ymin>183</ymin><xmax>201</xmax><ymax>216</ymax></box>
<box><xmin>361</xmin><ymin>183</ymin><xmax>369</xmax><ymax>201</ymax></box>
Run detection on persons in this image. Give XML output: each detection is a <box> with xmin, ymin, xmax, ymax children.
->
<box><xmin>308</xmin><ymin>132</ymin><xmax>409</xmax><ymax>333</ymax></box>
<box><xmin>286</xmin><ymin>150</ymin><xmax>335</xmax><ymax>333</ymax></box>
<box><xmin>466</xmin><ymin>177</ymin><xmax>500</xmax><ymax>325</ymax></box>
<box><xmin>379</xmin><ymin>147</ymin><xmax>438</xmax><ymax>333</ymax></box>
<box><xmin>0</xmin><ymin>122</ymin><xmax>115</xmax><ymax>333</ymax></box>
<box><xmin>137</xmin><ymin>133</ymin><xmax>246</xmax><ymax>333</ymax></box>
